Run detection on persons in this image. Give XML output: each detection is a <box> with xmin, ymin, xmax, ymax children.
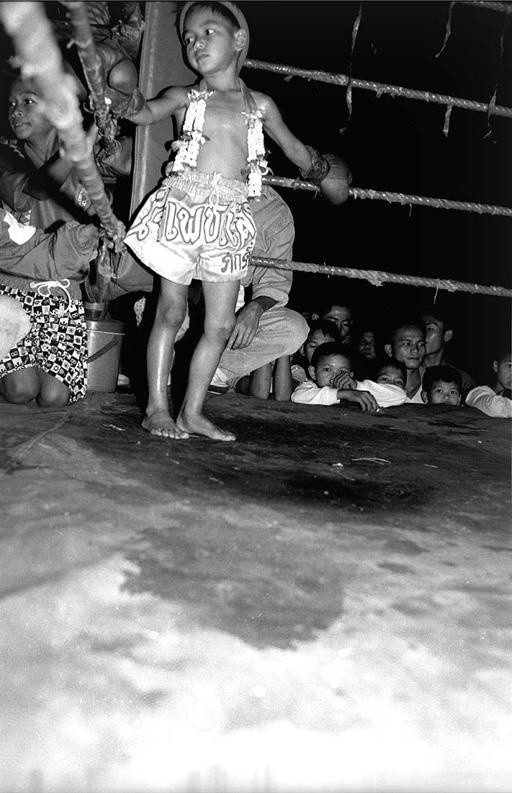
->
<box><xmin>1</xmin><ymin>74</ymin><xmax>121</xmax><ymax>408</ymax></box>
<box><xmin>204</xmin><ymin>179</ymin><xmax>310</xmax><ymax>396</ymax></box>
<box><xmin>110</xmin><ymin>1</ymin><xmax>353</xmax><ymax>443</ymax></box>
<box><xmin>233</xmin><ymin>298</ymin><xmax>511</xmax><ymax>422</ymax></box>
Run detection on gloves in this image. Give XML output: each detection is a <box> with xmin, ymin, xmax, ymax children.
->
<box><xmin>91</xmin><ymin>44</ymin><xmax>143</xmax><ymax>119</ymax></box>
<box><xmin>296</xmin><ymin>145</ymin><xmax>354</xmax><ymax>204</ymax></box>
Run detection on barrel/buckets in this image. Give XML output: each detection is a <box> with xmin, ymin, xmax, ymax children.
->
<box><xmin>83</xmin><ymin>318</ymin><xmax>127</xmax><ymax>394</ymax></box>
